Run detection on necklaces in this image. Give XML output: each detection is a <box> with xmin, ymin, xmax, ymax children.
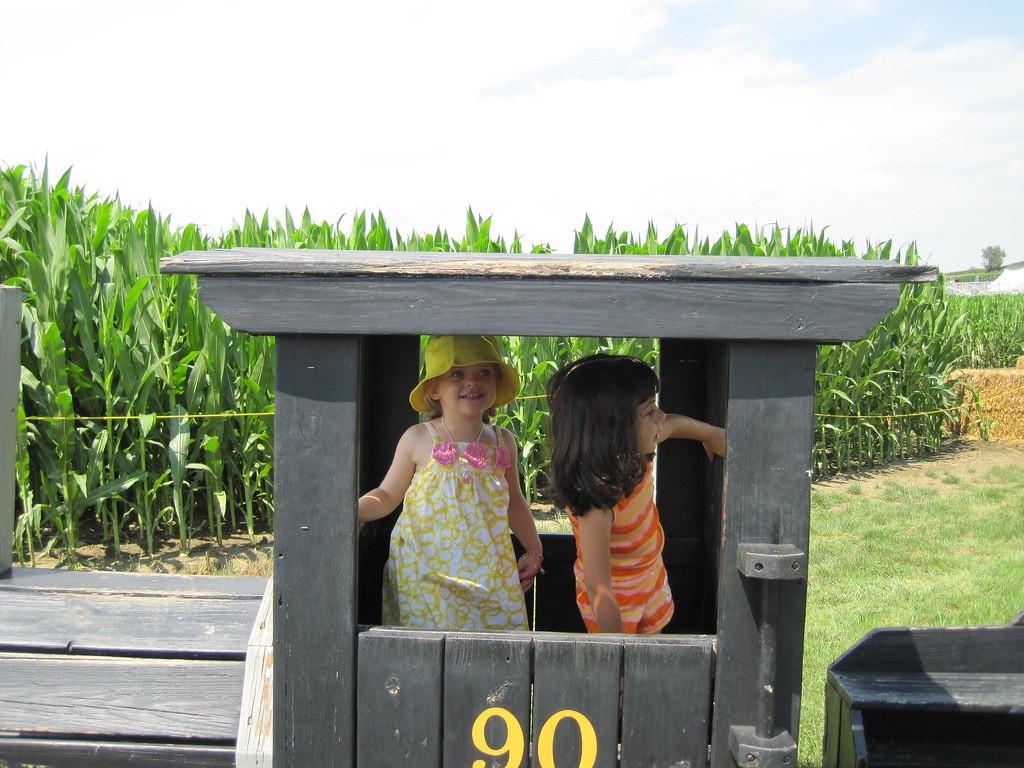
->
<box><xmin>442</xmin><ymin>417</ymin><xmax>483</xmax><ymax>482</ymax></box>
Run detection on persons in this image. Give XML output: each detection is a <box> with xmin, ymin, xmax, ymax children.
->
<box><xmin>548</xmin><ymin>353</ymin><xmax>726</xmax><ymax>717</ymax></box>
<box><xmin>358</xmin><ymin>334</ymin><xmax>544</xmax><ymax>630</ymax></box>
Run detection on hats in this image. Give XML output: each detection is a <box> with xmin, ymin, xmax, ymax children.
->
<box><xmin>409</xmin><ymin>335</ymin><xmax>519</xmax><ymax>413</ymax></box>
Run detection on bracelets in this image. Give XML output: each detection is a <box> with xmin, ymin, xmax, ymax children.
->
<box><xmin>519</xmin><ymin>552</ymin><xmax>543</xmax><ymax>562</ymax></box>
